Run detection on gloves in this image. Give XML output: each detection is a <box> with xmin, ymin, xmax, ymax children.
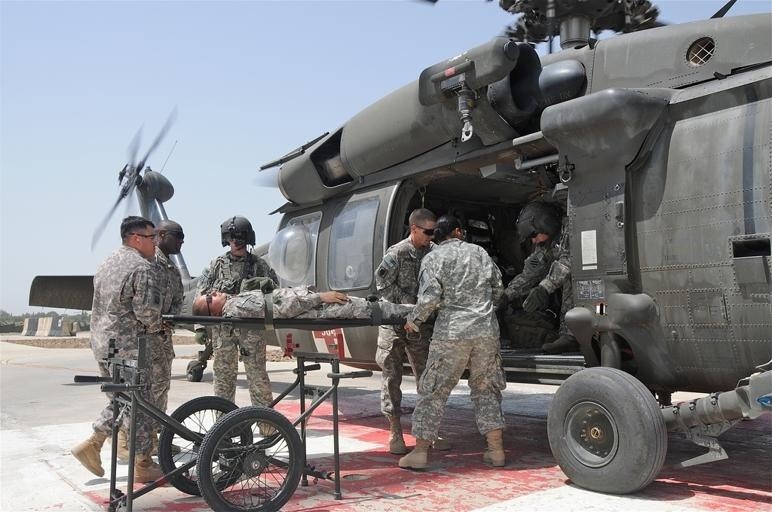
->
<box><xmin>522</xmin><ymin>287</ymin><xmax>548</xmax><ymax>312</ymax></box>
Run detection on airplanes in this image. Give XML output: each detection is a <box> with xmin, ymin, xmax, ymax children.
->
<box><xmin>91</xmin><ymin>0</ymin><xmax>763</xmax><ymax>496</ymax></box>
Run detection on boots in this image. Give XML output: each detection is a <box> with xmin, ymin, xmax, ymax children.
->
<box><xmin>72</xmin><ymin>432</ymin><xmax>181</xmax><ymax>484</ymax></box>
<box><xmin>484</xmin><ymin>429</ymin><xmax>506</xmax><ymax>467</ymax></box>
<box><xmin>399</xmin><ymin>439</ymin><xmax>431</xmax><ymax>468</ymax></box>
<box><xmin>432</xmin><ymin>435</ymin><xmax>451</xmax><ymax>450</ymax></box>
<box><xmin>390</xmin><ymin>423</ymin><xmax>406</xmax><ymax>454</ymax></box>
<box><xmin>542</xmin><ymin>335</ymin><xmax>579</xmax><ymax>354</ymax></box>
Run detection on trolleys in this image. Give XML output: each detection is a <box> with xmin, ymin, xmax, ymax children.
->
<box><xmin>75</xmin><ymin>337</ymin><xmax>381</xmax><ymax>512</ymax></box>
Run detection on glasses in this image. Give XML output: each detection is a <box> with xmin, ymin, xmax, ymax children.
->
<box><xmin>165</xmin><ymin>231</ymin><xmax>184</xmax><ymax>239</ymax></box>
<box><xmin>415</xmin><ymin>226</ymin><xmax>435</xmax><ymax>236</ymax></box>
<box><xmin>131</xmin><ymin>233</ymin><xmax>158</xmax><ymax>242</ymax></box>
<box><xmin>205</xmin><ymin>290</ymin><xmax>213</xmax><ymax>316</ymax></box>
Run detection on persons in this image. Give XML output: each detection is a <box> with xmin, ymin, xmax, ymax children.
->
<box><xmin>371</xmin><ymin>209</ymin><xmax>450</xmax><ymax>453</ymax></box>
<box><xmin>193</xmin><ymin>276</ymin><xmax>417</xmax><ymax>321</ymax></box>
<box><xmin>195</xmin><ymin>215</ymin><xmax>278</xmax><ymax>439</ymax></box>
<box><xmin>108</xmin><ymin>218</ymin><xmax>180</xmax><ymax>462</ymax></box>
<box><xmin>469</xmin><ymin>209</ymin><xmax>518</xmax><ymax>349</ymax></box>
<box><xmin>397</xmin><ymin>213</ymin><xmax>509</xmax><ymax>470</ymax></box>
<box><xmin>493</xmin><ymin>198</ymin><xmax>581</xmax><ymax>355</ymax></box>
<box><xmin>68</xmin><ymin>216</ymin><xmax>172</xmax><ymax>484</ymax></box>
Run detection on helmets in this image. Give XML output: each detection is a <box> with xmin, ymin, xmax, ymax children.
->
<box><xmin>516</xmin><ymin>202</ymin><xmax>561</xmax><ymax>245</ymax></box>
<box><xmin>220</xmin><ymin>217</ymin><xmax>255</xmax><ymax>247</ymax></box>
